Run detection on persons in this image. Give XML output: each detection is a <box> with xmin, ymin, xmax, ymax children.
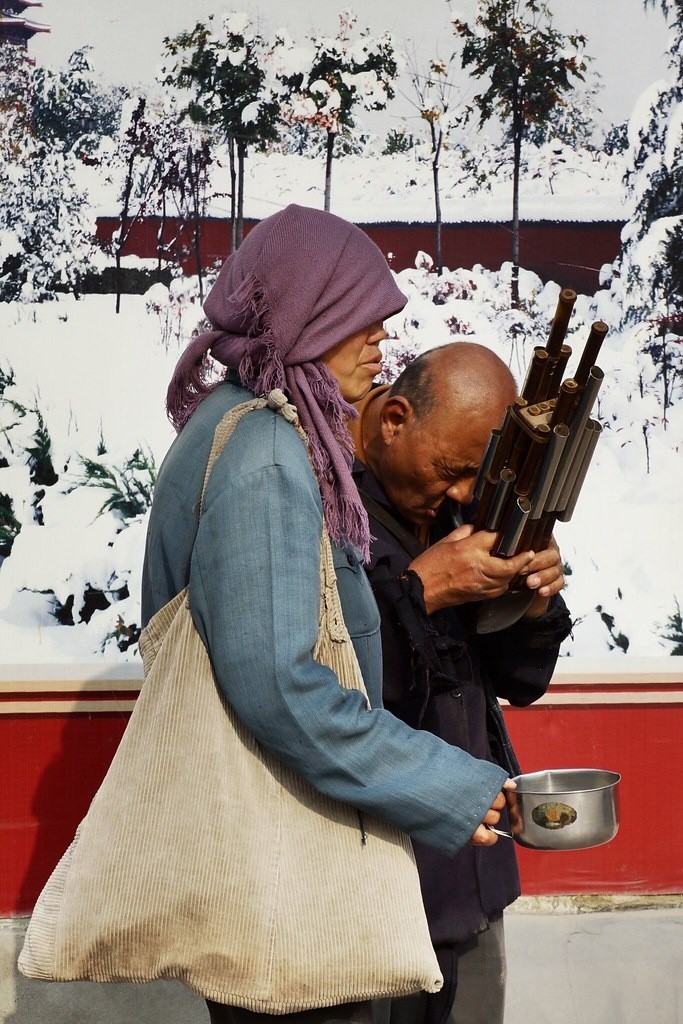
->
<box><xmin>140</xmin><ymin>204</ymin><xmax>518</xmax><ymax>1023</ymax></box>
<box><xmin>342</xmin><ymin>340</ymin><xmax>574</xmax><ymax>1022</ymax></box>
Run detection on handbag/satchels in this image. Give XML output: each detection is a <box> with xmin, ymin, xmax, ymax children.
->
<box><xmin>17</xmin><ymin>388</ymin><xmax>441</xmax><ymax>1014</ymax></box>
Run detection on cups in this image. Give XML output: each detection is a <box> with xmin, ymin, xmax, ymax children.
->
<box><xmin>487</xmin><ymin>768</ymin><xmax>619</xmax><ymax>849</ymax></box>
